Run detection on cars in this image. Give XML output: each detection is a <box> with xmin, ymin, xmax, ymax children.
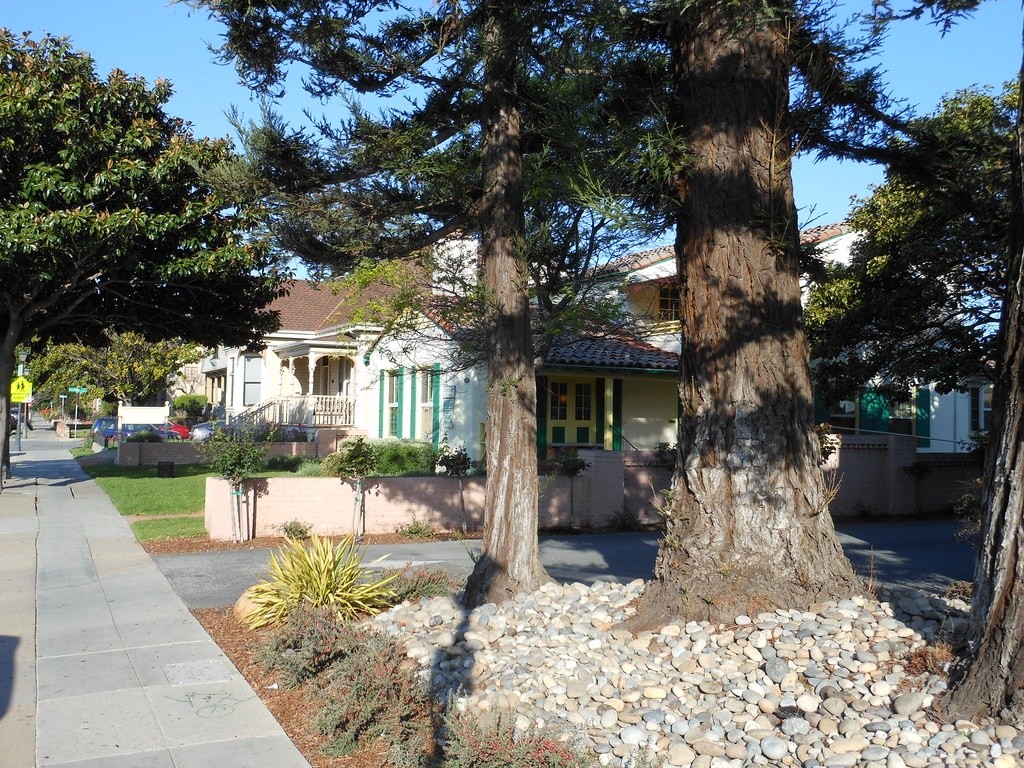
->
<box><xmin>91</xmin><ymin>416</ymin><xmax>181</xmax><ymax>441</ymax></box>
<box><xmin>152</xmin><ymin>420</ymin><xmax>190</xmax><ymax>439</ymax></box>
<box><xmin>10</xmin><ymin>410</ymin><xmax>18</xmax><ymax>419</ymax></box>
<box><xmin>191</xmin><ymin>420</ymin><xmax>225</xmax><ymax>441</ymax></box>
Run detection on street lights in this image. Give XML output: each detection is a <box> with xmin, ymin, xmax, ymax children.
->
<box><xmin>14</xmin><ymin>348</ymin><xmax>28</xmax><ymax>451</ymax></box>
